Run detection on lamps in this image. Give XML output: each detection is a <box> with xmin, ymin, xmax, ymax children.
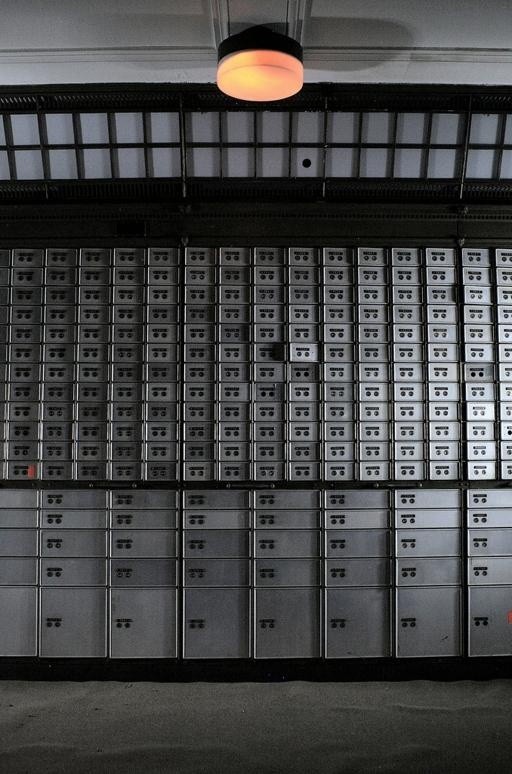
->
<box><xmin>210</xmin><ymin>0</ymin><xmax>313</xmax><ymax>102</ymax></box>
